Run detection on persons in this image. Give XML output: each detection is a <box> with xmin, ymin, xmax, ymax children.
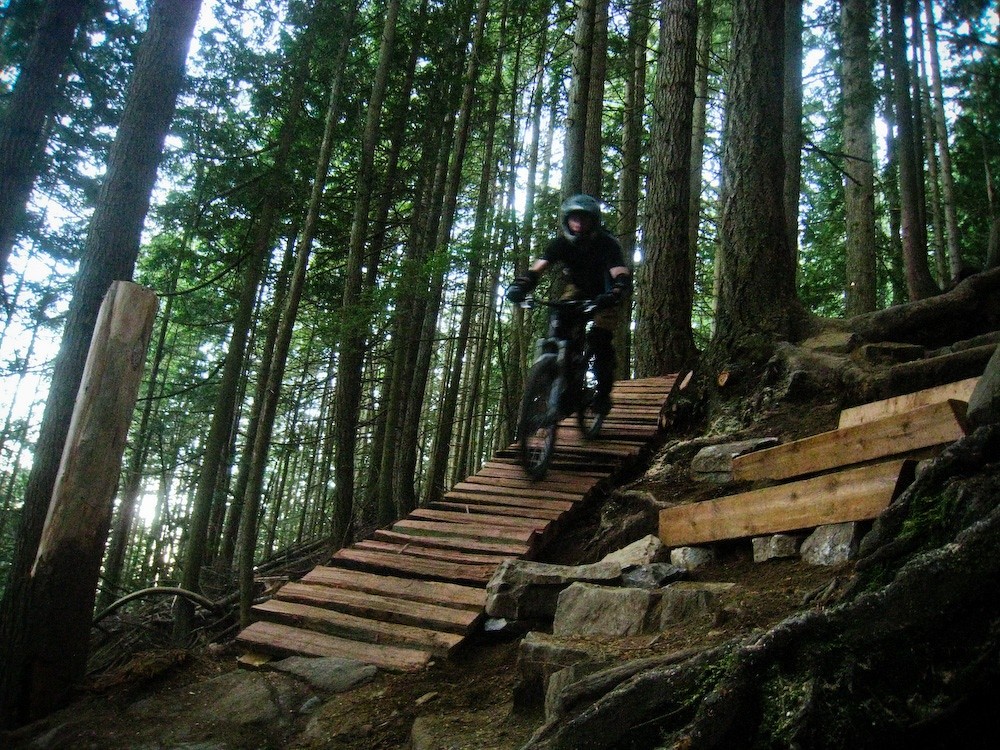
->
<box><xmin>506</xmin><ymin>194</ymin><xmax>634</xmax><ymax>429</ymax></box>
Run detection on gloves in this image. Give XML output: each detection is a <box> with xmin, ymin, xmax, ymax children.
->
<box><xmin>505</xmin><ymin>279</ymin><xmax>526</xmax><ymax>303</ymax></box>
<box><xmin>595</xmin><ymin>293</ymin><xmax>615</xmax><ymax>310</ymax></box>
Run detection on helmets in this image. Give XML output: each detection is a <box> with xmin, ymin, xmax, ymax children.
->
<box><xmin>560</xmin><ymin>195</ymin><xmax>600</xmax><ymax>245</ymax></box>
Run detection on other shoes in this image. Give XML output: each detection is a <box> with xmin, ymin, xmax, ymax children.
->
<box><xmin>590</xmin><ymin>390</ymin><xmax>613</xmax><ymax>415</ymax></box>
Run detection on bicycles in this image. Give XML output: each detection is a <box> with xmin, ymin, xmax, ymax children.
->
<box><xmin>508</xmin><ymin>290</ymin><xmax>605</xmax><ymax>481</ymax></box>
